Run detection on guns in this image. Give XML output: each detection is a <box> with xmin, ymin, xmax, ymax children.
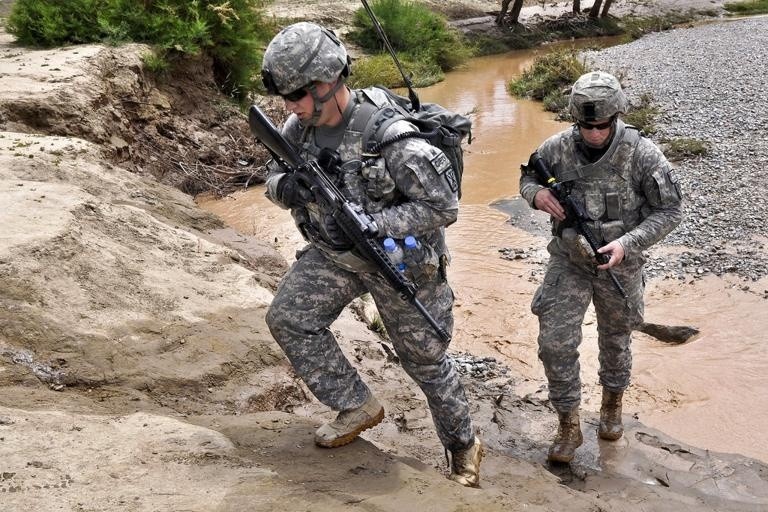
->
<box><xmin>247</xmin><ymin>104</ymin><xmax>453</xmax><ymax>342</ymax></box>
<box><xmin>529</xmin><ymin>152</ymin><xmax>629</xmax><ymax>301</ymax></box>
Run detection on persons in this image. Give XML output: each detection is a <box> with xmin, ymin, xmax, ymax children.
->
<box><xmin>261</xmin><ymin>22</ymin><xmax>484</xmax><ymax>487</ymax></box>
<box><xmin>518</xmin><ymin>71</ymin><xmax>681</xmax><ymax>463</ymax></box>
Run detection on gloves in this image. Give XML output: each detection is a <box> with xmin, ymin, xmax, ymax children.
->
<box><xmin>326</xmin><ymin>201</ymin><xmax>376</xmax><ymax>247</ymax></box>
<box><xmin>276</xmin><ymin>171</ymin><xmax>316</xmax><ymax>210</ymax></box>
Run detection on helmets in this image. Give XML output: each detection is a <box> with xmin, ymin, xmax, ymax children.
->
<box><xmin>567</xmin><ymin>72</ymin><xmax>631</xmax><ymax>121</ymax></box>
<box><xmin>261</xmin><ymin>22</ymin><xmax>352</xmax><ymax>96</ymax></box>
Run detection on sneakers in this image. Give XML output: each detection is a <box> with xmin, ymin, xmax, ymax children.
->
<box><xmin>448</xmin><ymin>436</ymin><xmax>482</xmax><ymax>489</ymax></box>
<box><xmin>315</xmin><ymin>390</ymin><xmax>385</xmax><ymax>448</ymax></box>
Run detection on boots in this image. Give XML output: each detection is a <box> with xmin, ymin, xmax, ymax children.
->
<box><xmin>599</xmin><ymin>387</ymin><xmax>624</xmax><ymax>440</ymax></box>
<box><xmin>547</xmin><ymin>405</ymin><xmax>584</xmax><ymax>463</ymax></box>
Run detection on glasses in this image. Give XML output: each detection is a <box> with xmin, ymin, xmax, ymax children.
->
<box><xmin>279</xmin><ymin>89</ymin><xmax>308</xmax><ymax>102</ymax></box>
<box><xmin>578</xmin><ymin>119</ymin><xmax>616</xmax><ymax>130</ymax></box>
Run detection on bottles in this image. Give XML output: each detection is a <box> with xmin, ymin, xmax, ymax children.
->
<box><xmin>404</xmin><ymin>236</ymin><xmax>417</xmax><ymax>249</ymax></box>
<box><xmin>383</xmin><ymin>238</ymin><xmax>406</xmax><ymax>274</ymax></box>
<box><xmin>397</xmin><ymin>264</ymin><xmax>407</xmax><ymax>273</ymax></box>
